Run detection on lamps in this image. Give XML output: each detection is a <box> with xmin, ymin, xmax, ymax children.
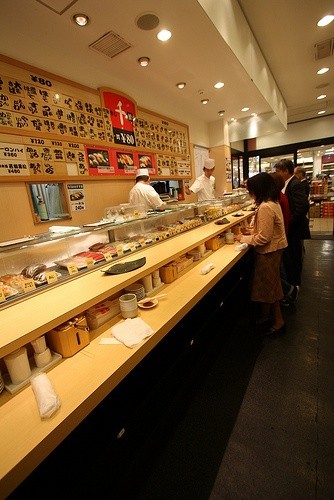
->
<box><xmin>75</xmin><ymin>15</ymin><xmax>88</xmax><ymax>26</ymax></box>
<box><xmin>140</xmin><ymin>58</ymin><xmax>149</xmax><ymax>67</ymax></box>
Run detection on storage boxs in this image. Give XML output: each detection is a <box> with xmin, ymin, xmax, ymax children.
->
<box><xmin>309</xmin><ymin>187</ymin><xmax>334</xmax><ymax>218</ymax></box>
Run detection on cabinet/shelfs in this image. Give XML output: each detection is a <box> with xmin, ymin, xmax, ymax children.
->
<box><xmin>0</xmin><ymin>194</ymin><xmax>252</xmax><ymax>500</ymax></box>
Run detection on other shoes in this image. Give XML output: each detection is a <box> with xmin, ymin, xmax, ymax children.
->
<box><xmin>289</xmin><ymin>285</ymin><xmax>299</xmax><ymax>303</ymax></box>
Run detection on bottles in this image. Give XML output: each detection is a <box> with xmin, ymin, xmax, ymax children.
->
<box><xmin>36</xmin><ymin>195</ymin><xmax>49</xmax><ymax>221</ymax></box>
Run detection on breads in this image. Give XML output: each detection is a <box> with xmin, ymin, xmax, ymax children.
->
<box><xmin>0</xmin><ymin>262</ymin><xmax>52</xmax><ymax>296</ymax></box>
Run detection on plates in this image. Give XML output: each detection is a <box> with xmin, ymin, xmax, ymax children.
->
<box><xmin>138</xmin><ymin>298</ymin><xmax>158</xmax><ymax>309</ymax></box>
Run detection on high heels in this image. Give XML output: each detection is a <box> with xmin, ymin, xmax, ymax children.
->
<box><xmin>265</xmin><ymin>320</ymin><xmax>288</xmax><ymax>338</ymax></box>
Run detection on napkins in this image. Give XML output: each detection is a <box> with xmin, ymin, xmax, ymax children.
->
<box><xmin>32</xmin><ymin>371</ymin><xmax>61</xmax><ymax>419</ymax></box>
<box><xmin>201</xmin><ymin>263</ymin><xmax>215</xmax><ymax>275</ymax></box>
<box><xmin>112</xmin><ymin>318</ymin><xmax>154</xmax><ymax>348</ymax></box>
<box><xmin>236</xmin><ymin>243</ymin><xmax>248</xmax><ymax>250</ymax></box>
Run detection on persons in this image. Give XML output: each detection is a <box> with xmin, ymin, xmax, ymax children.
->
<box><xmin>273</xmin><ymin>158</ymin><xmax>311</xmax><ymax>286</ymax></box>
<box><xmin>269</xmin><ymin>173</ymin><xmax>298</xmax><ymax>306</ymax></box>
<box><xmin>185</xmin><ymin>159</ymin><xmax>216</xmax><ymax>201</ymax></box>
<box><xmin>130</xmin><ymin>169</ymin><xmax>166</xmax><ymax>211</ymax></box>
<box><xmin>235</xmin><ymin>172</ymin><xmax>288</xmax><ymax>335</ymax></box>
<box><xmin>294</xmin><ymin>166</ymin><xmax>310</xmax><ymax>196</ymax></box>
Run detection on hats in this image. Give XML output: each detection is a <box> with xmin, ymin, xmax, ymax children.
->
<box><xmin>204</xmin><ymin>159</ymin><xmax>215</xmax><ymax>169</ymax></box>
<box><xmin>136</xmin><ymin>168</ymin><xmax>149</xmax><ymax>178</ymax></box>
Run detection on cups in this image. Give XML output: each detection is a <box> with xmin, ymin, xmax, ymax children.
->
<box><xmin>119</xmin><ymin>293</ymin><xmax>138</xmax><ymax>319</ymax></box>
<box><xmin>142</xmin><ymin>268</ymin><xmax>161</xmax><ymax>291</ymax></box>
<box><xmin>30</xmin><ymin>337</ymin><xmax>51</xmax><ymax>367</ymax></box>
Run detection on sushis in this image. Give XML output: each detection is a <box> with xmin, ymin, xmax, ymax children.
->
<box><xmin>117</xmin><ymin>154</ymin><xmax>152</xmax><ymax>167</ymax></box>
<box><xmin>87</xmin><ymin>152</ymin><xmax>109</xmax><ymax>167</ymax></box>
